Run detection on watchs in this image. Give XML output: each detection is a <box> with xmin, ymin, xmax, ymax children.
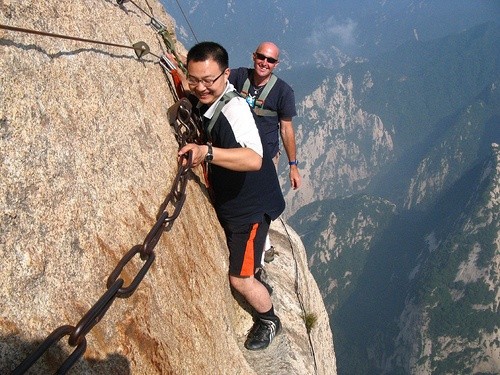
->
<box><xmin>204</xmin><ymin>146</ymin><xmax>214</xmax><ymax>163</ymax></box>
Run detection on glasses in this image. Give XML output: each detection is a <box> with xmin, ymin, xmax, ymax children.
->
<box><xmin>253</xmin><ymin>50</ymin><xmax>278</xmax><ymax>64</ymax></box>
<box><xmin>187</xmin><ymin>71</ymin><xmax>223</xmax><ymax>86</ymax></box>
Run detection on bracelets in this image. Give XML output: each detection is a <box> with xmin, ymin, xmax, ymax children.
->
<box><xmin>289</xmin><ymin>160</ymin><xmax>298</xmax><ymax>166</ymax></box>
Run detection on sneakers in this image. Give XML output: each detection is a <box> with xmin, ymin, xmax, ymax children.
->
<box><xmin>262</xmin><ymin>281</ymin><xmax>272</xmax><ymax>293</ymax></box>
<box><xmin>245</xmin><ymin>315</ymin><xmax>281</xmax><ymax>350</ymax></box>
<box><xmin>264</xmin><ymin>246</ymin><xmax>275</xmax><ymax>262</ymax></box>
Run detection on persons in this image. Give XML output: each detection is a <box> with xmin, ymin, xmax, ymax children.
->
<box><xmin>171</xmin><ymin>42</ymin><xmax>301</xmax><ymax>283</ymax></box>
<box><xmin>162</xmin><ymin>41</ymin><xmax>286</xmax><ymax>351</ymax></box>
